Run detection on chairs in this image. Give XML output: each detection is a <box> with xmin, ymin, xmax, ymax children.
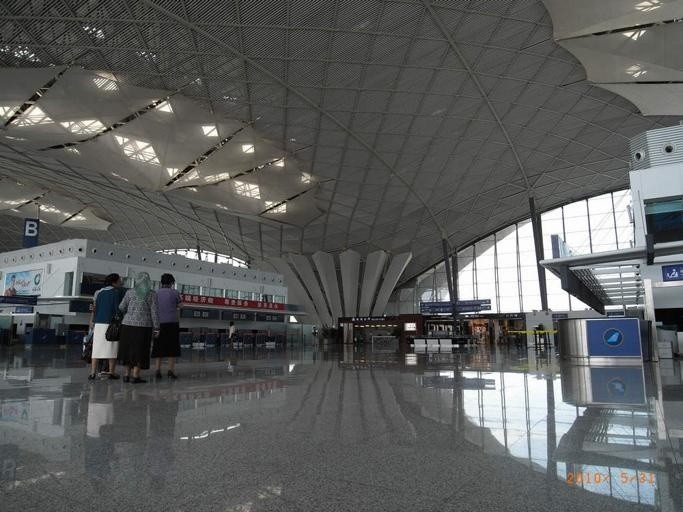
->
<box><xmin>414</xmin><ymin>338</ymin><xmax>453</xmax><ymax>354</ymax></box>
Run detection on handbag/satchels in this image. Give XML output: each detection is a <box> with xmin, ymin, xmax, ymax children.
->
<box><xmin>105</xmin><ymin>311</ymin><xmax>122</xmax><ymax>341</ymax></box>
<box><xmin>81</xmin><ymin>334</ymin><xmax>93</xmax><ymax>364</ymax></box>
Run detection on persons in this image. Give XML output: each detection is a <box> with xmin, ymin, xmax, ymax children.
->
<box><xmin>86</xmin><ymin>273</ymin><xmax>125</xmax><ymax>379</ymax></box>
<box><xmin>3</xmin><ymin>275</ymin><xmax>16</xmax><ymax>296</ymax></box>
<box><xmin>151</xmin><ymin>273</ymin><xmax>181</xmax><ymax>379</ymax></box>
<box><xmin>87</xmin><ymin>303</ymin><xmax>109</xmax><ymax>377</ymax></box>
<box><xmin>228</xmin><ymin>321</ymin><xmax>236</xmax><ymax>349</ymax></box>
<box><xmin>82</xmin><ymin>330</ymin><xmax>87</xmax><ymax>351</ymax></box>
<box><xmin>117</xmin><ymin>271</ymin><xmax>159</xmax><ymax>383</ymax></box>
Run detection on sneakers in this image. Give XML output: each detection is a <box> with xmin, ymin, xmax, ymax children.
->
<box><xmin>88</xmin><ymin>371</ymin><xmax>178</xmax><ymax>383</ymax></box>
